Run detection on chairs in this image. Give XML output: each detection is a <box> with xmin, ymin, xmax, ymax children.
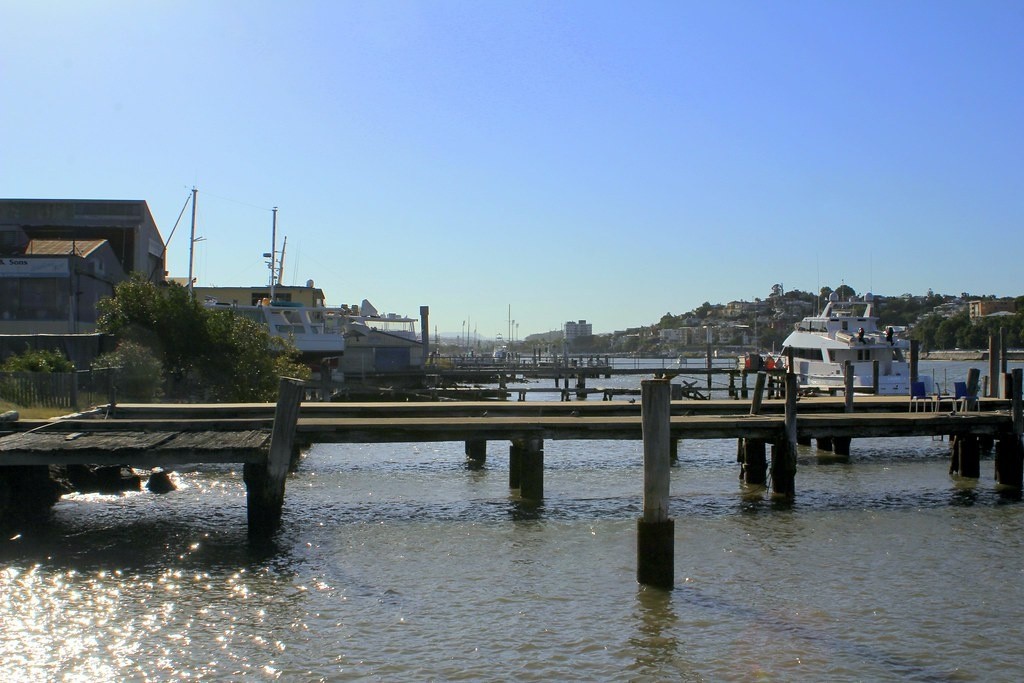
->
<box><xmin>909</xmin><ymin>382</ymin><xmax>934</xmax><ymax>414</ymax></box>
<box><xmin>953</xmin><ymin>382</ymin><xmax>980</xmax><ymax>415</ymax></box>
<box><xmin>934</xmin><ymin>382</ymin><xmax>957</xmax><ymax>414</ymax></box>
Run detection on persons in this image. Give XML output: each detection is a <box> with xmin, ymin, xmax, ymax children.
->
<box><xmin>469</xmin><ymin>351</ymin><xmax>473</xmax><ymax>359</ymax></box>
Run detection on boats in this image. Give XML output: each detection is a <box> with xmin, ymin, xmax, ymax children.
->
<box><xmin>137</xmin><ymin>186</ymin><xmax>346</xmax><ymax>357</ymax></box>
<box><xmin>780</xmin><ymin>251</ymin><xmax>934</xmax><ymax>396</ymax></box>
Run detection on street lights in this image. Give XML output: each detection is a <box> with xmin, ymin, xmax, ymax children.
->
<box><xmin>462</xmin><ymin>320</ymin><xmax>466</xmax><ymax>347</ymax></box>
<box><xmin>512</xmin><ymin>320</ymin><xmax>519</xmax><ymax>354</ymax></box>
